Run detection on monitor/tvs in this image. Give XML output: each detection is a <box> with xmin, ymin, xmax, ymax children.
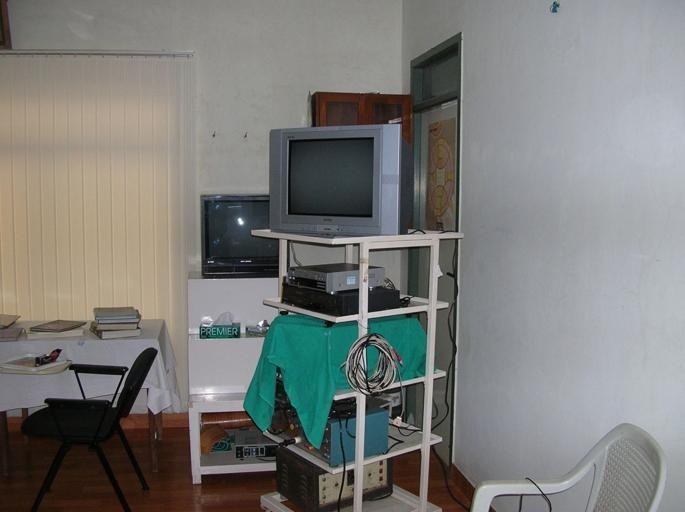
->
<box><xmin>269</xmin><ymin>123</ymin><xmax>407</xmax><ymax>236</ymax></box>
<box><xmin>199</xmin><ymin>194</ymin><xmax>279</xmax><ymax>278</ymax></box>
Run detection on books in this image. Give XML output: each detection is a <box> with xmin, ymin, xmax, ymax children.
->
<box><xmin>0</xmin><ymin>307</ymin><xmax>141</xmax><ymax>375</ymax></box>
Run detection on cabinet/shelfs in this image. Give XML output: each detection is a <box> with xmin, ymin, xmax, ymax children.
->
<box><xmin>250</xmin><ymin>228</ymin><xmax>465</xmax><ymax>510</ymax></box>
<box><xmin>183</xmin><ymin>268</ymin><xmax>299</xmax><ymax>487</ymax></box>
<box><xmin>310</xmin><ymin>91</ymin><xmax>413</xmax><ymax>149</ymax></box>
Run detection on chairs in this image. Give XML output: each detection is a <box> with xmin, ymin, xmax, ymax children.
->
<box><xmin>470</xmin><ymin>422</ymin><xmax>669</xmax><ymax>512</ymax></box>
<box><xmin>18</xmin><ymin>347</ymin><xmax>160</xmax><ymax>510</ymax></box>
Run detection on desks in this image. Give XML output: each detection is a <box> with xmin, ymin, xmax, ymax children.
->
<box><xmin>0</xmin><ymin>319</ymin><xmax>178</xmax><ymax>474</ymax></box>
